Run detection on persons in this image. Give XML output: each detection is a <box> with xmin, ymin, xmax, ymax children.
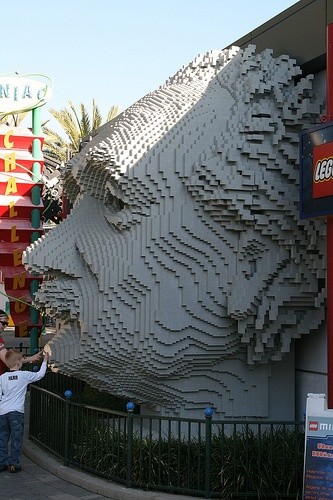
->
<box><xmin>22</xmin><ymin>44</ymin><xmax>327</xmax><ymax>419</ymax></box>
<box><xmin>0</xmin><ymin>352</ymin><xmax>49</xmax><ymax>473</ymax></box>
<box><xmin>0</xmin><ymin>309</ymin><xmax>44</xmax><ymax>376</ymax></box>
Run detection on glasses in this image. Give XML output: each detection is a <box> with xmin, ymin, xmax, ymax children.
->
<box><xmin>0</xmin><ymin>321</ymin><xmax>8</xmax><ymax>327</ymax></box>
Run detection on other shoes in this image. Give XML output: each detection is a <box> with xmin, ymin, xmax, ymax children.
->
<box><xmin>9</xmin><ymin>465</ymin><xmax>22</xmax><ymax>473</ymax></box>
<box><xmin>0</xmin><ymin>466</ymin><xmax>7</xmax><ymax>471</ymax></box>
